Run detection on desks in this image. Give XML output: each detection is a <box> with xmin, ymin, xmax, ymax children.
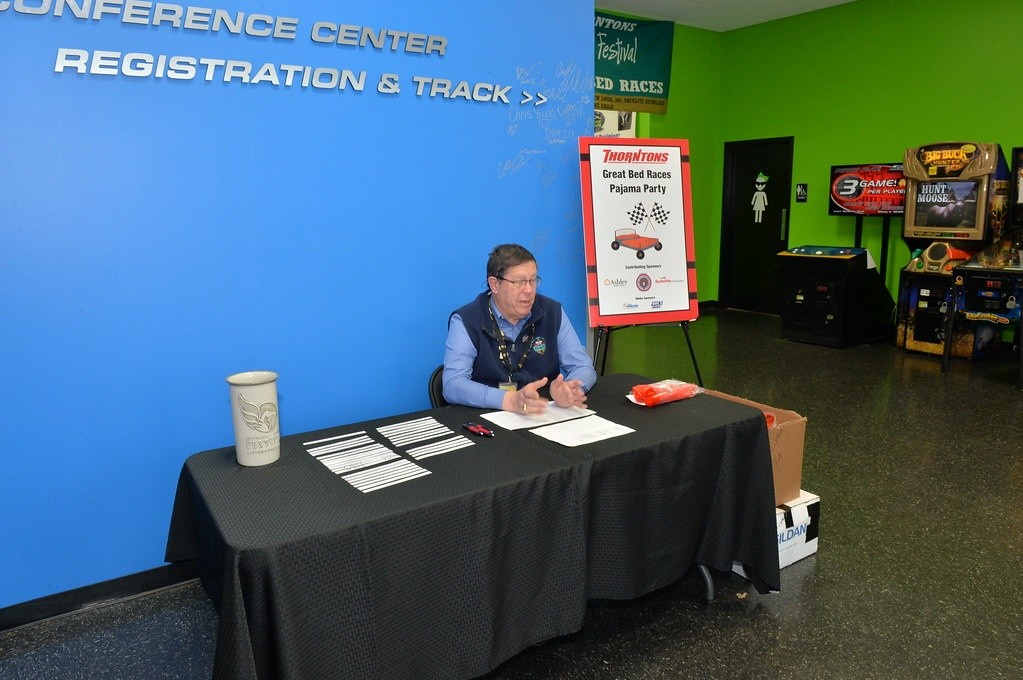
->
<box><xmin>940</xmin><ymin>267</ymin><xmax>1023</xmax><ymax>375</ymax></box>
<box><xmin>164</xmin><ymin>372</ymin><xmax>781</xmax><ymax>680</ymax></box>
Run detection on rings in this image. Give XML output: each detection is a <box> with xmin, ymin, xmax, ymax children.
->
<box><xmin>523</xmin><ymin>404</ymin><xmax>527</xmax><ymax>410</ymax></box>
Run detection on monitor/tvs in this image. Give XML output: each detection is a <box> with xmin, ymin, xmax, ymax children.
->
<box><xmin>915</xmin><ymin>180</ymin><xmax>978</xmax><ymax>229</ymax></box>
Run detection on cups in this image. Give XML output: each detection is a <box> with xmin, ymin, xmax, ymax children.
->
<box><xmin>227</xmin><ymin>371</ymin><xmax>281</xmax><ymax>467</ymax></box>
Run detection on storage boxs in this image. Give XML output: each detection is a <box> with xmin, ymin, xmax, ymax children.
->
<box><xmin>704</xmin><ymin>390</ymin><xmax>808</xmax><ymax>507</ymax></box>
<box><xmin>732</xmin><ymin>490</ymin><xmax>820</xmax><ymax>578</ymax></box>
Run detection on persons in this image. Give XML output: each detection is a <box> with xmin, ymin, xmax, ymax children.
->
<box><xmin>442</xmin><ymin>244</ymin><xmax>597</xmax><ymax>415</ymax></box>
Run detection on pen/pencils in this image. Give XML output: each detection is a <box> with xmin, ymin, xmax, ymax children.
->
<box><xmin>463</xmin><ymin>420</ymin><xmax>495</xmax><ymax>437</ymax></box>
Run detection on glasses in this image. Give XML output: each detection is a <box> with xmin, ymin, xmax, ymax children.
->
<box><xmin>495</xmin><ymin>276</ymin><xmax>541</xmax><ymax>290</ymax></box>
